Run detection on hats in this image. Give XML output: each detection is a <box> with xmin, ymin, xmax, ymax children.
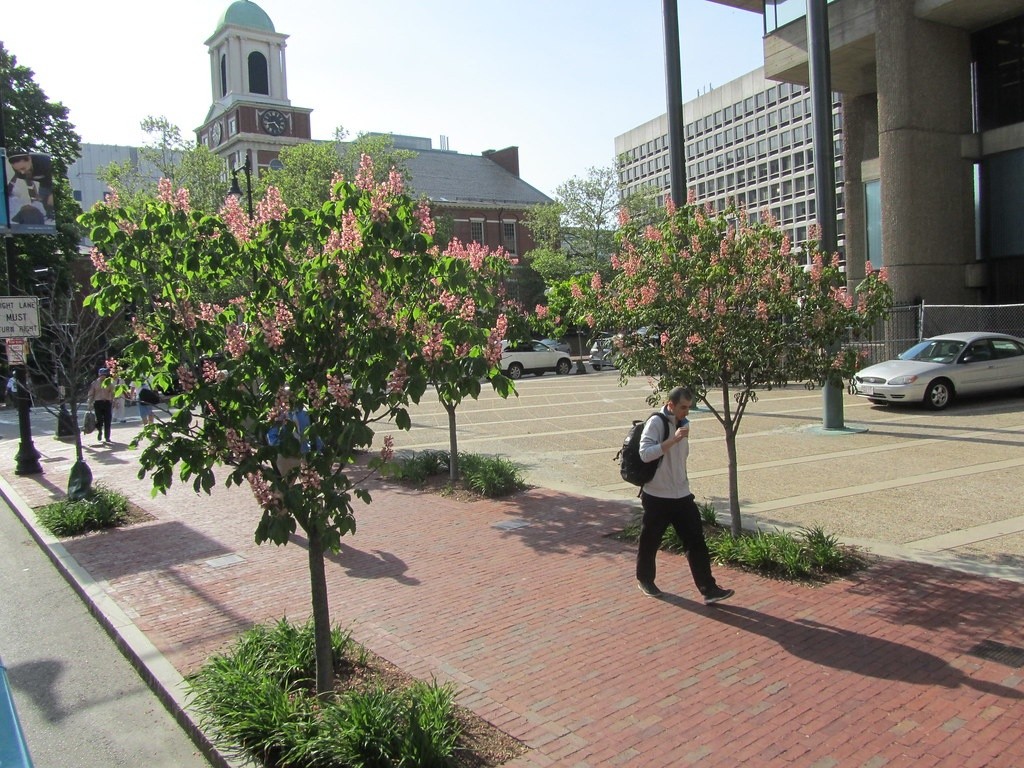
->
<box><xmin>99</xmin><ymin>368</ymin><xmax>107</xmax><ymax>376</ymax></box>
<box><xmin>12</xmin><ymin>370</ymin><xmax>16</xmax><ymax>375</ymax></box>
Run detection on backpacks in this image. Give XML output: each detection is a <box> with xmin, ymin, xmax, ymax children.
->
<box><xmin>612</xmin><ymin>411</ymin><xmax>670</xmax><ymax>499</ymax></box>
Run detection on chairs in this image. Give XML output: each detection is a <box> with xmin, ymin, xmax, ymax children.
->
<box><xmin>948</xmin><ymin>346</ymin><xmax>959</xmax><ymax>354</ymax></box>
<box><xmin>974</xmin><ymin>345</ymin><xmax>987</xmax><ymax>353</ymax></box>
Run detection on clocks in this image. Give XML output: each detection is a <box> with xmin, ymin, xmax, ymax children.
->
<box><xmin>260</xmin><ymin>109</ymin><xmax>287</xmax><ymax>136</ymax></box>
<box><xmin>211</xmin><ymin>120</ymin><xmax>222</xmax><ymax>147</ymax></box>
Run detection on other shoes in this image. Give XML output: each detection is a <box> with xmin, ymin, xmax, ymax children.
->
<box><xmin>29</xmin><ymin>410</ymin><xmax>31</xmax><ymax>412</ymax></box>
<box><xmin>120</xmin><ymin>420</ymin><xmax>126</xmax><ymax>423</ymax></box>
<box><xmin>16</xmin><ymin>411</ymin><xmax>19</xmax><ymax>415</ymax></box>
<box><xmin>98</xmin><ymin>431</ymin><xmax>102</xmax><ymax>440</ymax></box>
<box><xmin>106</xmin><ymin>437</ymin><xmax>110</xmax><ymax>442</ymax></box>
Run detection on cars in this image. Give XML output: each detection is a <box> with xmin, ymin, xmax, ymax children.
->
<box><xmin>532</xmin><ymin>335</ymin><xmax>573</xmax><ymax>357</ymax></box>
<box><xmin>588</xmin><ymin>324</ymin><xmax>661</xmax><ymax>371</ymax></box>
<box><xmin>492</xmin><ymin>338</ymin><xmax>574</xmax><ymax>381</ymax></box>
<box><xmin>853</xmin><ymin>331</ymin><xmax>1024</xmax><ymax>411</ymax></box>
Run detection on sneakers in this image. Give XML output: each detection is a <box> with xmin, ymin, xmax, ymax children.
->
<box><xmin>704</xmin><ymin>585</ymin><xmax>734</xmax><ymax>604</ymax></box>
<box><xmin>638</xmin><ymin>580</ymin><xmax>663</xmax><ymax>598</ymax></box>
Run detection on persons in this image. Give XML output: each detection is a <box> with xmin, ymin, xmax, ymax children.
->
<box><xmin>201</xmin><ymin>370</ymin><xmax>233</xmax><ymax>463</ymax></box>
<box><xmin>4</xmin><ymin>371</ymin><xmax>18</xmax><ymax>416</ymax></box>
<box><xmin>635</xmin><ymin>387</ymin><xmax>735</xmax><ymax>604</ymax></box>
<box><xmin>87</xmin><ymin>368</ymin><xmax>119</xmax><ymax>442</ymax></box>
<box><xmin>266</xmin><ymin>387</ymin><xmax>322</xmax><ymax>515</ymax></box>
<box><xmin>111</xmin><ymin>373</ymin><xmax>128</xmax><ymax>422</ymax></box>
<box><xmin>129</xmin><ymin>372</ymin><xmax>154</xmax><ymax>425</ymax></box>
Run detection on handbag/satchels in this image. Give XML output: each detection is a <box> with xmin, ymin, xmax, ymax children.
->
<box><xmin>84</xmin><ymin>410</ymin><xmax>96</xmax><ymax>435</ymax></box>
<box><xmin>139</xmin><ymin>388</ymin><xmax>159</xmax><ymax>404</ymax></box>
